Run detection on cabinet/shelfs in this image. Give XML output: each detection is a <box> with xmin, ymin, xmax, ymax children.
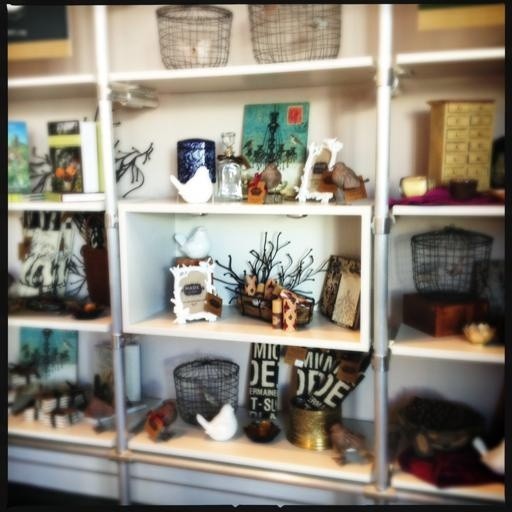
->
<box><xmin>427</xmin><ymin>98</ymin><xmax>496</xmax><ymax>192</ymax></box>
<box><xmin>8</xmin><ymin>3</ymin><xmax>506</xmax><ymax>505</ymax></box>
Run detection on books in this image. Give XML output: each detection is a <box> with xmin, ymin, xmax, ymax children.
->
<box><xmin>43</xmin><ymin>193</ymin><xmax>107</xmax><ymax>203</ymax></box>
<box><xmin>45</xmin><ymin>119</ymin><xmax>105</xmax><ymax>193</ymax></box>
